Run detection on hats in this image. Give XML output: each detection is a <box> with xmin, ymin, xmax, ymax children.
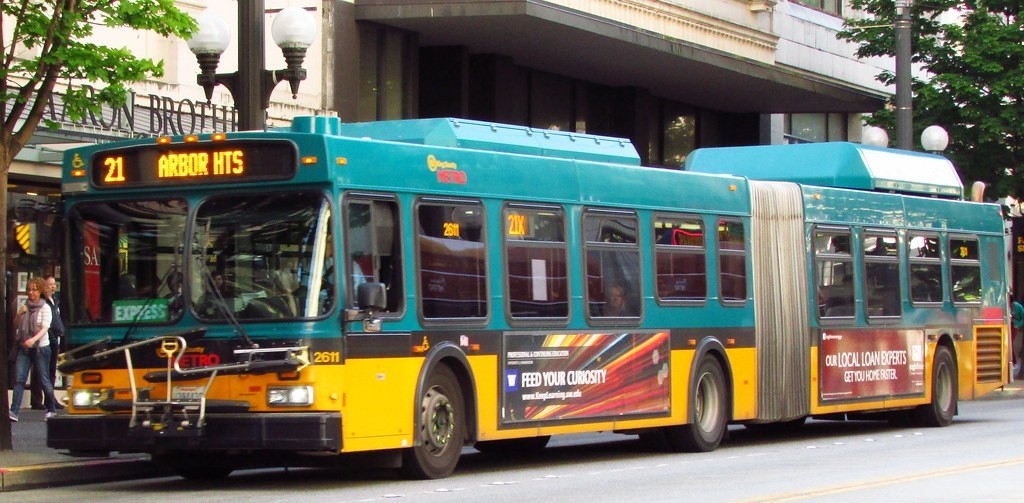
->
<box><xmin>305</xmin><ymin>219</ymin><xmax>331</xmax><ymax>245</ymax></box>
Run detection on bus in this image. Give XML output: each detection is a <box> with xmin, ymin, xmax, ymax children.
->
<box><xmin>8</xmin><ymin>115</ymin><xmax>1014</xmax><ymax>482</ymax></box>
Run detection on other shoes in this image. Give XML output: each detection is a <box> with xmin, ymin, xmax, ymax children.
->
<box><xmin>9</xmin><ymin>409</ymin><xmax>18</xmax><ymax>421</ymax></box>
<box><xmin>43</xmin><ymin>411</ymin><xmax>57</xmax><ymax>421</ymax></box>
<box><xmin>44</xmin><ymin>402</ymin><xmax>64</xmax><ymax>409</ymax></box>
<box><xmin>31</xmin><ymin>405</ymin><xmax>47</xmax><ymax>409</ymax></box>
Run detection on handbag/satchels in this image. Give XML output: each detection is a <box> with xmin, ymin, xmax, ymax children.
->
<box><xmin>8</xmin><ymin>342</ymin><xmax>18</xmax><ymax>362</ymax></box>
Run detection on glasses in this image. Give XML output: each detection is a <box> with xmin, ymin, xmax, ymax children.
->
<box><xmin>26</xmin><ymin>287</ymin><xmax>39</xmax><ymax>292</ymax></box>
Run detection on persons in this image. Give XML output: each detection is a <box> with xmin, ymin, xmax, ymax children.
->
<box><xmin>1010</xmin><ymin>295</ymin><xmax>1024</xmax><ymax>370</ymax></box>
<box><xmin>30</xmin><ymin>275</ymin><xmax>67</xmax><ymax>409</ymax></box>
<box><xmin>9</xmin><ymin>277</ymin><xmax>57</xmax><ymax>421</ymax></box>
<box><xmin>167</xmin><ymin>271</ymin><xmax>186</xmax><ymax>312</ymax></box>
<box><xmin>602</xmin><ymin>284</ymin><xmax>628</xmax><ymax>316</ymax></box>
<box><xmin>210</xmin><ymin>272</ymin><xmax>229</xmax><ymax>299</ymax></box>
<box><xmin>306</xmin><ymin>235</ymin><xmax>366</xmax><ymax>313</ymax></box>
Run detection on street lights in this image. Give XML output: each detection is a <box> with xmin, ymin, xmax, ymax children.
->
<box><xmin>188</xmin><ymin>5</ymin><xmax>315</xmax><ymax>132</ymax></box>
<box><xmin>860</xmin><ymin>125</ymin><xmax>950</xmax><ymax>156</ymax></box>
